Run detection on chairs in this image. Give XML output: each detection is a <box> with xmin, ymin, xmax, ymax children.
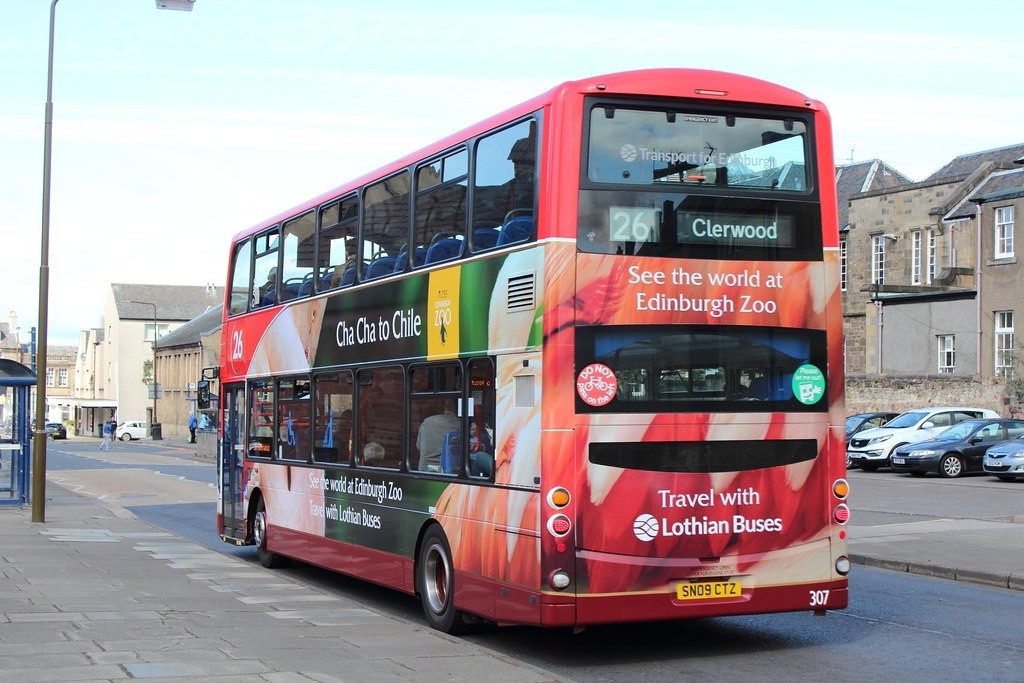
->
<box><xmin>744</xmin><ymin>371</ymin><xmax>794</xmax><ymax>401</ymax></box>
<box><xmin>982</xmin><ymin>428</ymin><xmax>991</xmax><ymax>442</ymax></box>
<box><xmin>439</xmin><ymin>433</ymin><xmax>492</xmax><ymax>473</ymax></box>
<box><xmin>263</xmin><ymin>207</ymin><xmax>532</xmax><ymax>306</ymax></box>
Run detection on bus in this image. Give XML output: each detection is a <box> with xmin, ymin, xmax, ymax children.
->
<box><xmin>196</xmin><ymin>68</ymin><xmax>851</xmax><ymax>638</ymax></box>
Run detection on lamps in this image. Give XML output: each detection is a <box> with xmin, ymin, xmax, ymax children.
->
<box><xmin>882</xmin><ymin>233</ymin><xmax>899</xmax><ymax>240</ymax></box>
<box><xmin>925</xmin><ymin>223</ymin><xmax>944</xmax><ymax>235</ymax></box>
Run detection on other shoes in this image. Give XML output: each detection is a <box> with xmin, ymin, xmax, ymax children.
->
<box><xmin>191</xmin><ymin>441</ymin><xmax>197</xmax><ymax>443</ymax></box>
<box><xmin>99</xmin><ymin>446</ymin><xmax>103</xmax><ymax>449</ymax></box>
<box><xmin>106</xmin><ymin>449</ymin><xmax>112</xmax><ymax>451</ymax></box>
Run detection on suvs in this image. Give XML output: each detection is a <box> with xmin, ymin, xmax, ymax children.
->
<box><xmin>30</xmin><ymin>418</ymin><xmax>49</xmax><ymax>439</ymax></box>
<box><xmin>116</xmin><ymin>421</ymin><xmax>146</xmax><ymax>442</ymax></box>
<box><xmin>847</xmin><ymin>407</ymin><xmax>1002</xmax><ymax>472</ymax></box>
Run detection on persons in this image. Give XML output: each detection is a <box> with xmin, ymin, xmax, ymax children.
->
<box><xmin>188</xmin><ymin>411</ymin><xmax>197</xmax><ymax>443</ymax></box>
<box><xmin>261</xmin><ymin>267</ymin><xmax>277</xmax><ymax>299</ymax></box>
<box><xmin>362</xmin><ymin>442</ymin><xmax>394</xmax><ymax>467</ymax></box>
<box><xmin>330</xmin><ymin>239</ymin><xmax>356</xmax><ymax>288</ymax></box>
<box><xmin>415</xmin><ymin>398</ymin><xmax>461</xmax><ymax>471</ymax></box>
<box><xmin>110</xmin><ymin>416</ymin><xmax>117</xmax><ymax>441</ymax></box>
<box><xmin>100</xmin><ymin>420</ymin><xmax>111</xmax><ymax>450</ymax></box>
<box><xmin>200</xmin><ymin>413</ymin><xmax>213</xmax><ymax>431</ymax></box>
<box><xmin>472</xmin><ymin>452</ymin><xmax>493</xmax><ymax>477</ymax></box>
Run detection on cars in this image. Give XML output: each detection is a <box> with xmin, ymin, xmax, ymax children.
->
<box><xmin>844</xmin><ymin>412</ymin><xmax>901</xmax><ymax>470</ymax></box>
<box><xmin>982</xmin><ymin>433</ymin><xmax>1024</xmax><ymax>483</ymax></box>
<box><xmin>890</xmin><ymin>418</ymin><xmax>1024</xmax><ymax>478</ymax></box>
<box><xmin>46</xmin><ymin>423</ymin><xmax>66</xmax><ymax>440</ymax></box>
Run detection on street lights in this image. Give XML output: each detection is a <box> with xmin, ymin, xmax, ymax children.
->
<box><xmin>130</xmin><ymin>301</ymin><xmax>157</xmax><ymax>428</ymax></box>
<box><xmin>31</xmin><ymin>0</ymin><xmax>199</xmax><ymax>524</ymax></box>
